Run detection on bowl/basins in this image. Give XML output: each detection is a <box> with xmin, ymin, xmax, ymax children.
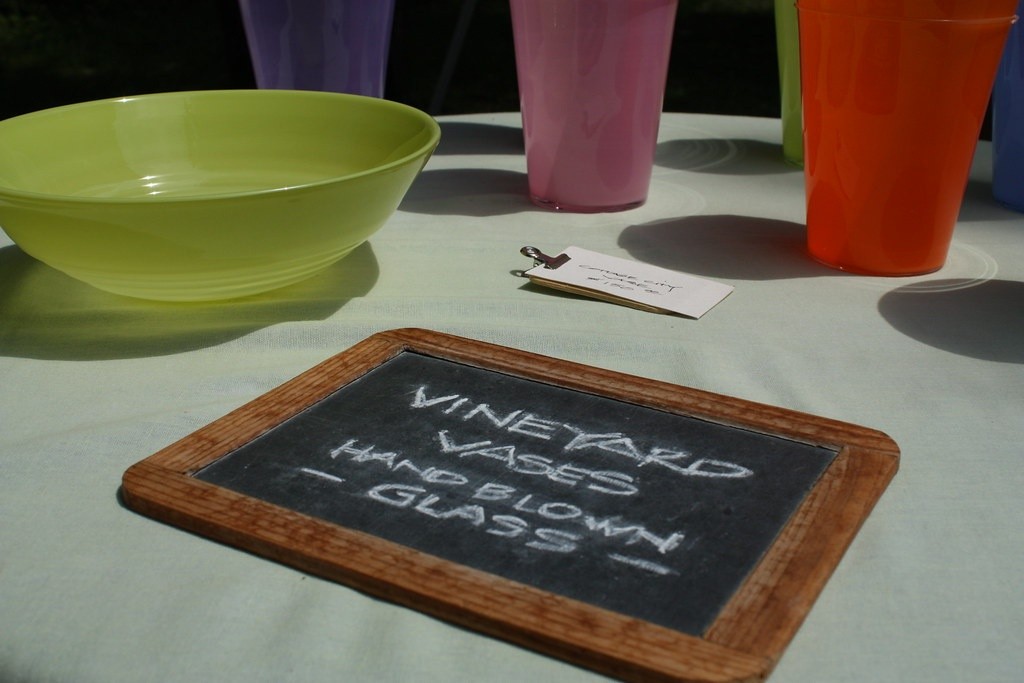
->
<box><xmin>0</xmin><ymin>88</ymin><xmax>442</xmax><ymax>304</ymax></box>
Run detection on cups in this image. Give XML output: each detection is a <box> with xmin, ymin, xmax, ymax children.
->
<box><xmin>507</xmin><ymin>0</ymin><xmax>680</xmax><ymax>212</ymax></box>
<box><xmin>773</xmin><ymin>0</ymin><xmax>806</xmax><ymax>166</ymax></box>
<box><xmin>990</xmin><ymin>0</ymin><xmax>1024</xmax><ymax>215</ymax></box>
<box><xmin>239</xmin><ymin>0</ymin><xmax>392</xmax><ymax>100</ymax></box>
<box><xmin>798</xmin><ymin>0</ymin><xmax>1020</xmax><ymax>275</ymax></box>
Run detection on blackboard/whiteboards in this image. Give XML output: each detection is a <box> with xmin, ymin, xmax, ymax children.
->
<box><xmin>120</xmin><ymin>325</ymin><xmax>903</xmax><ymax>683</ymax></box>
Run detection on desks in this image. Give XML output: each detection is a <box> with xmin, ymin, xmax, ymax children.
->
<box><xmin>0</xmin><ymin>111</ymin><xmax>1024</xmax><ymax>683</ymax></box>
